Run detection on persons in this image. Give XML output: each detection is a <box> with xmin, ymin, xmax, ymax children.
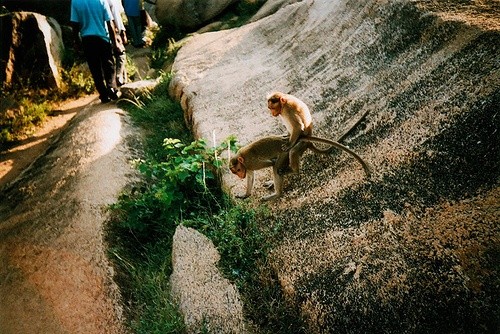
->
<box><xmin>70</xmin><ymin>0</ymin><xmax>130</xmax><ymax>103</ymax></box>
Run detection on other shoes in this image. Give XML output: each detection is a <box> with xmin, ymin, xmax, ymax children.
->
<box><xmin>133</xmin><ymin>44</ymin><xmax>144</xmax><ymax>48</ymax></box>
<box><xmin>101</xmin><ymin>88</ymin><xmax>119</xmax><ymax>103</ymax></box>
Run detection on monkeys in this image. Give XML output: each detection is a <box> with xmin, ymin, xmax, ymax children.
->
<box><xmin>229</xmin><ymin>135</ymin><xmax>371</xmax><ymax>198</ymax></box>
<box><xmin>268</xmin><ymin>93</ymin><xmax>370</xmax><ymax>175</ymax></box>
